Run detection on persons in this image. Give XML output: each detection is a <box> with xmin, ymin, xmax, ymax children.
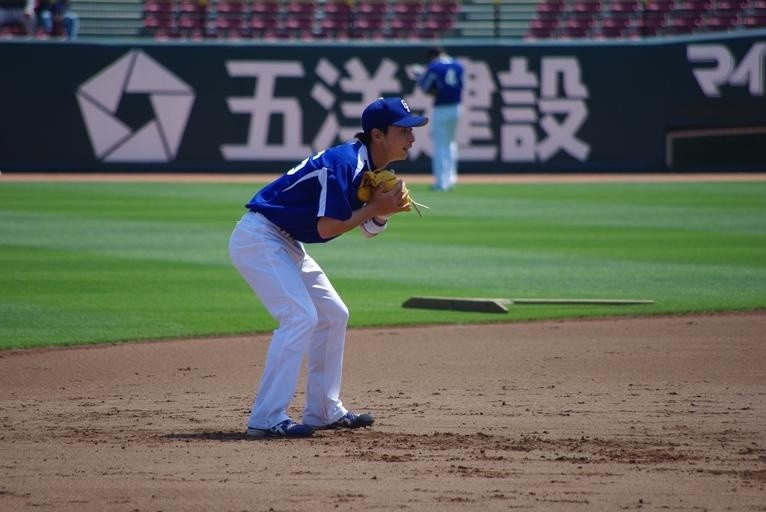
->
<box><xmin>227</xmin><ymin>96</ymin><xmax>430</xmax><ymax>437</ymax></box>
<box><xmin>411</xmin><ymin>43</ymin><xmax>466</xmax><ymax>192</ymax></box>
<box><xmin>1</xmin><ymin>1</ymin><xmax>82</xmax><ymax>44</ymax></box>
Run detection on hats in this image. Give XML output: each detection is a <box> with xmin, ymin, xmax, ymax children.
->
<box><xmin>361</xmin><ymin>95</ymin><xmax>429</xmax><ymax>132</ymax></box>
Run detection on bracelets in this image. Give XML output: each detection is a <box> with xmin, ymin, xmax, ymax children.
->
<box><xmin>362</xmin><ymin>218</ymin><xmax>390</xmax><ymax>237</ymax></box>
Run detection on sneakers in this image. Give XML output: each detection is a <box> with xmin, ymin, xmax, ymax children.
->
<box><xmin>314</xmin><ymin>409</ymin><xmax>376</xmax><ymax>430</ymax></box>
<box><xmin>246</xmin><ymin>415</ymin><xmax>317</xmax><ymax>438</ymax></box>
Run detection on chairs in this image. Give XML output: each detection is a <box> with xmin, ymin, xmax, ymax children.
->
<box><xmin>0</xmin><ymin>0</ymin><xmax>766</xmax><ymax>47</ymax></box>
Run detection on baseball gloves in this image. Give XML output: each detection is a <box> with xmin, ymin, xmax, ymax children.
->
<box><xmin>357</xmin><ymin>170</ymin><xmax>429</xmax><ymax>218</ymax></box>
